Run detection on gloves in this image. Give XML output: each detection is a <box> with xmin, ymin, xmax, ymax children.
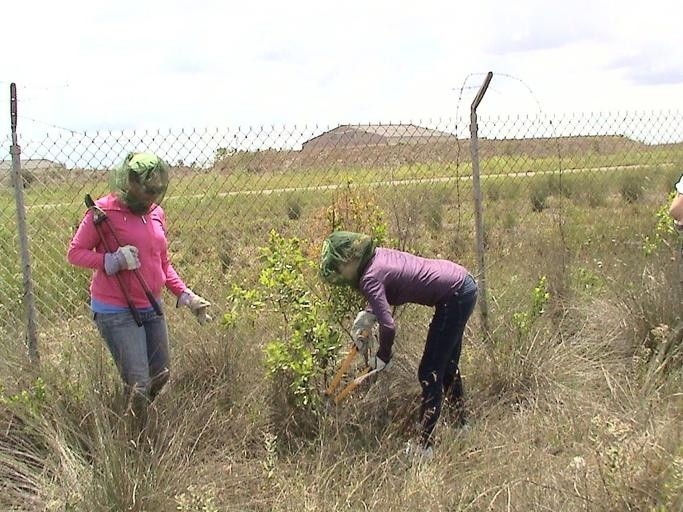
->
<box><xmin>176</xmin><ymin>287</ymin><xmax>212</xmax><ymax>326</ymax></box>
<box><xmin>104</xmin><ymin>245</ymin><xmax>141</xmax><ymax>275</ymax></box>
<box><xmin>367</xmin><ymin>356</ymin><xmax>386</xmax><ymax>373</ymax></box>
<box><xmin>349</xmin><ymin>311</ymin><xmax>376</xmax><ymax>355</ymax></box>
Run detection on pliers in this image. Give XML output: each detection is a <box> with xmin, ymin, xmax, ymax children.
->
<box><xmin>83</xmin><ymin>192</ymin><xmax>163</xmax><ymax>327</ymax></box>
<box><xmin>320</xmin><ymin>331</ymin><xmax>395</xmax><ymax>411</ymax></box>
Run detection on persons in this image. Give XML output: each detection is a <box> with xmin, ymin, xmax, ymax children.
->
<box><xmin>318</xmin><ymin>229</ymin><xmax>479</xmax><ymax>463</ymax></box>
<box><xmin>668</xmin><ymin>173</ymin><xmax>683</xmax><ymax>234</ymax></box>
<box><xmin>65</xmin><ymin>151</ymin><xmax>213</xmax><ymax>453</ymax></box>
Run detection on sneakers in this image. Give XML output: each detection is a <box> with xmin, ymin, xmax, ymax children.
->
<box><xmin>407</xmin><ymin>447</ymin><xmax>432</xmax><ymax>465</ymax></box>
<box><xmin>449</xmin><ymin>426</ymin><xmax>469</xmax><ymax>441</ymax></box>
